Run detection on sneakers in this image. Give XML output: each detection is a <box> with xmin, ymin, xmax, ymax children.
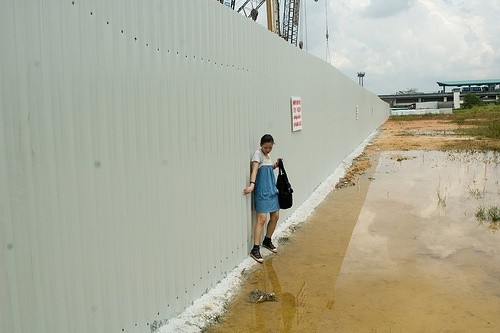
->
<box><xmin>263</xmin><ymin>238</ymin><xmax>278</xmax><ymax>253</ymax></box>
<box><xmin>250</xmin><ymin>247</ymin><xmax>264</xmax><ymax>263</ymax></box>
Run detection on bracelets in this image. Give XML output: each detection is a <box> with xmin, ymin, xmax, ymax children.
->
<box><xmin>250</xmin><ymin>182</ymin><xmax>255</xmax><ymax>185</ymax></box>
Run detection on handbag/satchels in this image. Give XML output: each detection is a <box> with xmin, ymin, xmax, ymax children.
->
<box><xmin>276</xmin><ymin>159</ymin><xmax>293</xmax><ymax>209</ymax></box>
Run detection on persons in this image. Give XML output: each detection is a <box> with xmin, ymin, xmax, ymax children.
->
<box><xmin>244</xmin><ymin>134</ymin><xmax>283</xmax><ymax>262</ymax></box>
<box><xmin>254</xmin><ymin>258</ymin><xmax>291</xmax><ymax>333</ymax></box>
